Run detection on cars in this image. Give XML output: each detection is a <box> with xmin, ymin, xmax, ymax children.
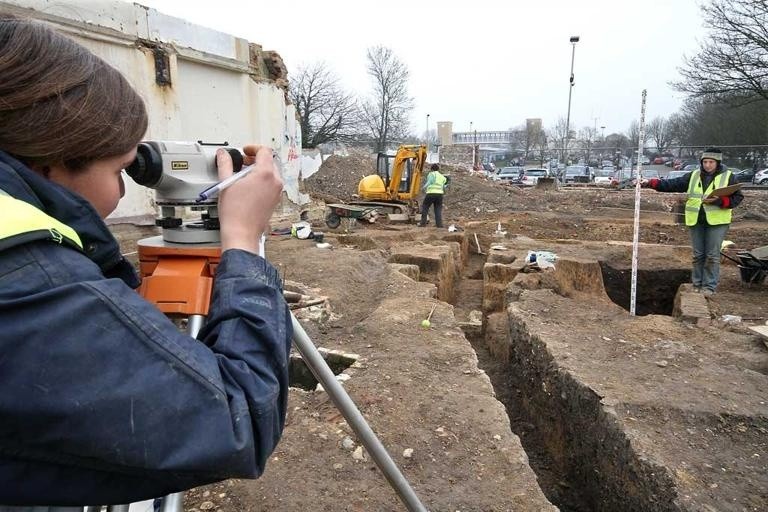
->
<box><xmin>494</xmin><ymin>149</ymin><xmax>767</xmax><ymax>188</ymax></box>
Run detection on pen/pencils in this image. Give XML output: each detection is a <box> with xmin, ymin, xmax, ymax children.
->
<box><xmin>196</xmin><ymin>152</ymin><xmax>278</xmax><ymax>203</ymax></box>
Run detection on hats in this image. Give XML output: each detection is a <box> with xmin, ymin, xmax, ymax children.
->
<box><xmin>700</xmin><ymin>148</ymin><xmax>722</xmax><ymax>168</ymax></box>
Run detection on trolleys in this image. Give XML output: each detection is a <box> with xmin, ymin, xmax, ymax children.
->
<box><xmin>720</xmin><ymin>244</ymin><xmax>768</xmax><ymax>286</ymax></box>
<box><xmin>321</xmin><ymin>203</ymin><xmax>379</xmax><ymax>228</ymax></box>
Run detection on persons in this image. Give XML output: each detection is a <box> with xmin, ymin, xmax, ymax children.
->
<box><xmin>632</xmin><ymin>147</ymin><xmax>743</xmax><ymax>296</ymax></box>
<box><xmin>0</xmin><ymin>19</ymin><xmax>294</xmax><ymax>512</ymax></box>
<box><xmin>416</xmin><ymin>163</ymin><xmax>446</xmax><ymax>227</ymax></box>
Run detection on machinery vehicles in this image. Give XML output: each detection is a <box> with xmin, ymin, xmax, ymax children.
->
<box><xmin>343</xmin><ymin>143</ymin><xmax>427</xmax><ymax>224</ymax></box>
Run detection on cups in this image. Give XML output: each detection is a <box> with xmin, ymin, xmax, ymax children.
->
<box><xmin>314</xmin><ymin>232</ymin><xmax>324</xmax><ymax>243</ymax></box>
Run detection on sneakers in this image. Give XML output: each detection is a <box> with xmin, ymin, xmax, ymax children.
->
<box><xmin>695</xmin><ymin>286</ymin><xmax>714</xmax><ymax>298</ymax></box>
<box><xmin>418</xmin><ymin>223</ymin><xmax>426</xmax><ymax>227</ymax></box>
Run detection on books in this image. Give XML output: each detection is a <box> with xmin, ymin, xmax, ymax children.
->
<box><xmin>705</xmin><ymin>183</ymin><xmax>742</xmax><ymax>199</ymax></box>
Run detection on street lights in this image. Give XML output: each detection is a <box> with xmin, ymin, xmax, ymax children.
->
<box><xmin>599</xmin><ymin>126</ymin><xmax>607</xmax><ymax>141</ymax></box>
<box><xmin>425</xmin><ymin>113</ymin><xmax>431</xmax><ymax>156</ymax></box>
<box><xmin>559</xmin><ymin>34</ymin><xmax>581</xmax><ymax>184</ymax></box>
<box><xmin>469</xmin><ymin>121</ymin><xmax>473</xmax><ymax>133</ymax></box>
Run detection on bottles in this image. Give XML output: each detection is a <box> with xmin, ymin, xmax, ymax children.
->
<box><xmin>530</xmin><ymin>253</ymin><xmax>536</xmax><ymax>263</ymax></box>
<box><xmin>498</xmin><ymin>222</ymin><xmax>501</xmax><ymax>230</ymax></box>
